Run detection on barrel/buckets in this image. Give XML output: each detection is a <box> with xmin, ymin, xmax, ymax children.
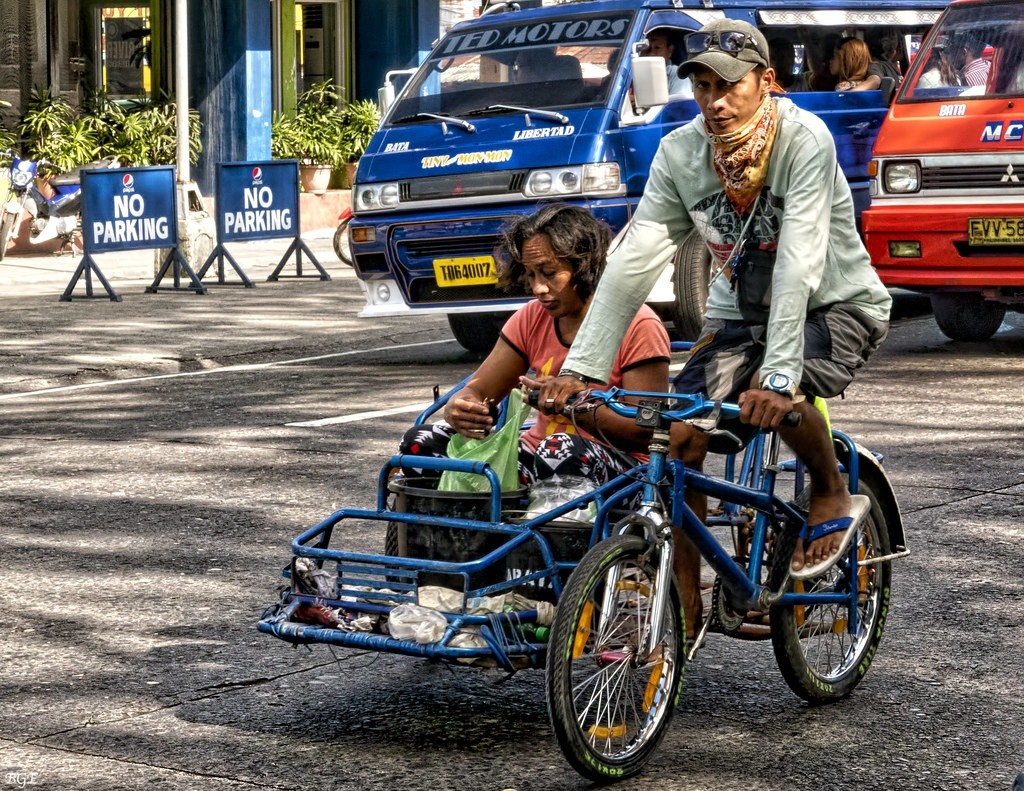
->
<box><xmin>498</xmin><ymin>508</ymin><xmax>613</xmax><ymax>620</ymax></box>
<box><xmin>387</xmin><ymin>477</ymin><xmax>531</xmax><ymax>601</ymax></box>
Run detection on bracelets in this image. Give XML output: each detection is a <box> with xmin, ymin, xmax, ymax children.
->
<box><xmin>558</xmin><ymin>369</ymin><xmax>589</xmax><ymax>386</ymax></box>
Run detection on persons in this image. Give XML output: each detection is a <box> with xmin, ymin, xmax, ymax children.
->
<box><xmin>768</xmin><ymin>25</ymin><xmax>903</xmax><ymax>92</ymax></box>
<box><xmin>915</xmin><ymin>29</ymin><xmax>992</xmax><ymax>89</ymax></box>
<box><xmin>539</xmin><ymin>19</ymin><xmax>893</xmax><ymax>648</ymax></box>
<box><xmin>399</xmin><ymin>202</ymin><xmax>671</xmax><ymax>489</ymax></box>
<box><xmin>512</xmin><ymin>49</ymin><xmax>555</xmax><ymax>83</ymax></box>
<box><xmin>640</xmin><ymin>28</ymin><xmax>695</xmax><ymax>100</ymax></box>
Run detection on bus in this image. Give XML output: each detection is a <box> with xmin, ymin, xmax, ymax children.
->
<box><xmin>862</xmin><ymin>1</ymin><xmax>1024</xmax><ymax>341</ymax></box>
<box><xmin>334</xmin><ymin>0</ymin><xmax>947</xmax><ymax>357</ymax></box>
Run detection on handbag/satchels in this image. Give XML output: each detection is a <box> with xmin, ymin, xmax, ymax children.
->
<box><xmin>731</xmin><ymin>243</ymin><xmax>786</xmax><ymax>312</ymax></box>
<box><xmin>438</xmin><ymin>387</ymin><xmax>527</xmax><ymax>493</ymax></box>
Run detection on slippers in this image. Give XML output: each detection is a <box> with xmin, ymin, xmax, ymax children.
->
<box><xmin>788</xmin><ymin>495</ymin><xmax>872</xmax><ymax>579</ymax></box>
<box><xmin>682</xmin><ymin>628</ymin><xmax>707</xmax><ymax>659</ymax></box>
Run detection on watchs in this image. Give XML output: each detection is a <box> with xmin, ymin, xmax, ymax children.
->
<box><xmin>763</xmin><ymin>372</ymin><xmax>797</xmax><ymax>401</ymax></box>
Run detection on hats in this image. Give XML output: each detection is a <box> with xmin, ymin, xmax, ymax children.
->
<box><xmin>677</xmin><ymin>18</ymin><xmax>769</xmax><ymax>82</ymax></box>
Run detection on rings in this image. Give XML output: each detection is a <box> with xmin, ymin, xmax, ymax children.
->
<box><xmin>545</xmin><ymin>399</ymin><xmax>554</xmax><ymax>402</ymax></box>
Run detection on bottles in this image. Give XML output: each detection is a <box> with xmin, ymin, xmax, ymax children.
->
<box><xmin>503</xmin><ymin>619</ymin><xmax>549</xmax><ymax>642</ymax></box>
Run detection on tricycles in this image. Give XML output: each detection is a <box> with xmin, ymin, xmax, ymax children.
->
<box><xmin>257</xmin><ymin>360</ymin><xmax>908</xmax><ymax>783</ymax></box>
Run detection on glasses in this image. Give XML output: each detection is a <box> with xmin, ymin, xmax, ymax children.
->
<box><xmin>683</xmin><ymin>30</ymin><xmax>769</xmax><ymax>71</ymax></box>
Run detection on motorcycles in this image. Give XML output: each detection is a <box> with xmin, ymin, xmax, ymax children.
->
<box><xmin>0</xmin><ymin>150</ymin><xmax>119</xmax><ymax>258</ymax></box>
<box><xmin>334</xmin><ymin>210</ymin><xmax>354</xmax><ymax>267</ymax></box>
